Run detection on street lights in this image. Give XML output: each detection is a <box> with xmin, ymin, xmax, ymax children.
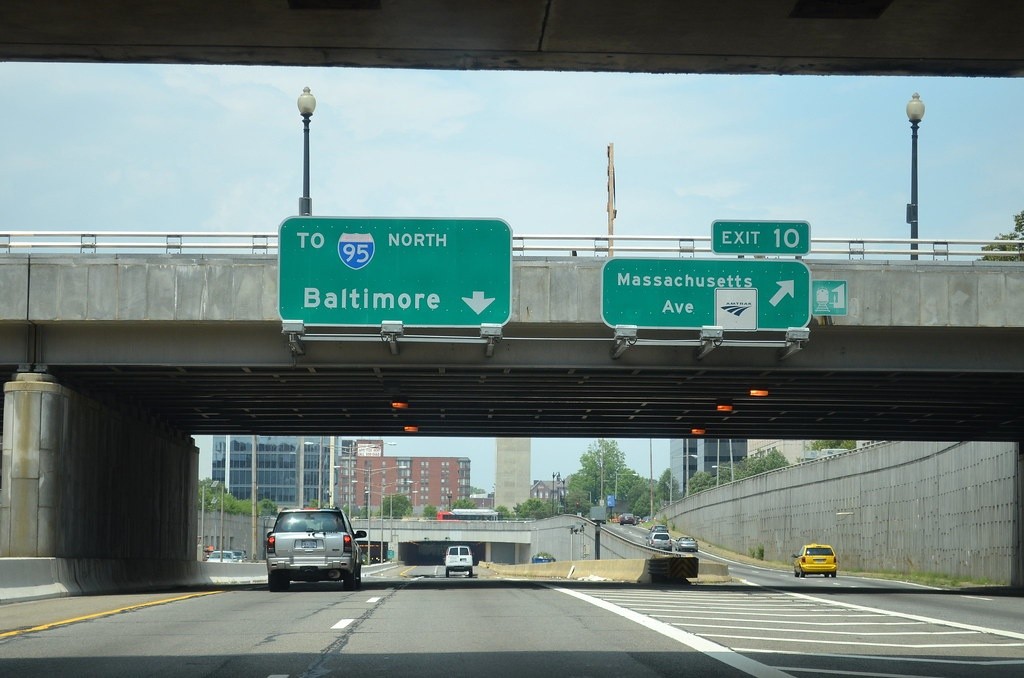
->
<box><xmin>305</xmin><ymin>437</ymin><xmax>397</xmax><ymax>531</ymax></box>
<box><xmin>711</xmin><ymin>465</ymin><xmax>735</xmax><ymax>482</ymax></box>
<box><xmin>552</xmin><ymin>471</ymin><xmax>560</xmax><ymax>517</ymax></box>
<box><xmin>365</xmin><ymin>488</ymin><xmax>418</xmax><ymax>563</ymax></box>
<box><xmin>615</xmin><ymin>473</ymin><xmax>639</xmax><ymax>499</ymax></box>
<box><xmin>351</xmin><ymin>477</ymin><xmax>413</xmax><ymax>565</ymax></box>
<box><xmin>904</xmin><ymin>91</ymin><xmax>925</xmax><ymax>259</ymax></box>
<box><xmin>557</xmin><ymin>478</ymin><xmax>567</xmax><ymax>514</ymax></box>
<box><xmin>669</xmin><ymin>454</ymin><xmax>698</xmax><ymax>505</ymax></box>
<box><xmin>332</xmin><ymin>464</ymin><xmax>408</xmax><ymax>567</ymax></box>
<box><xmin>298</xmin><ymin>86</ymin><xmax>318</xmax><ymax>217</ymax></box>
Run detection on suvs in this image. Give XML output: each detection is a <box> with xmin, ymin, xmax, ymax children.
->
<box><xmin>645</xmin><ymin>533</ymin><xmax>672</xmax><ymax>551</ymax></box>
<box><xmin>268</xmin><ymin>506</ymin><xmax>367</xmax><ymax>591</ymax></box>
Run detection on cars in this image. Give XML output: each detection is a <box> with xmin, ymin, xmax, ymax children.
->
<box><xmin>649</xmin><ymin>525</ymin><xmax>669</xmax><ymax>535</ymax></box>
<box><xmin>619</xmin><ymin>513</ymin><xmax>636</xmax><ymax>526</ymax></box>
<box><xmin>228</xmin><ymin>550</ymin><xmax>247</xmax><ymax>562</ymax></box>
<box><xmin>206</xmin><ymin>551</ymin><xmax>238</xmax><ymax>563</ymax></box>
<box><xmin>674</xmin><ymin>536</ymin><xmax>698</xmax><ymax>552</ymax></box>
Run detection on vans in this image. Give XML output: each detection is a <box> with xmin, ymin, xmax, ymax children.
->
<box><xmin>445</xmin><ymin>546</ymin><xmax>474</xmax><ymax>579</ymax></box>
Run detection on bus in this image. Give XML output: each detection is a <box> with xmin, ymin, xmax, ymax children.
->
<box><xmin>436</xmin><ymin>508</ymin><xmax>499</xmax><ymax>521</ymax></box>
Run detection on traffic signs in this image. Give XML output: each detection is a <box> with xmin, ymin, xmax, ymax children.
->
<box><xmin>708</xmin><ymin>218</ymin><xmax>813</xmax><ymax>260</ymax></box>
<box><xmin>276</xmin><ymin>212</ymin><xmax>514</xmax><ymax>335</ymax></box>
<box><xmin>598</xmin><ymin>257</ymin><xmax>814</xmax><ymax>337</ymax></box>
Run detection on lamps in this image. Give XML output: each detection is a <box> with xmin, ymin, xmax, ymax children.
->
<box><xmin>692</xmin><ymin>429</ymin><xmax>705</xmax><ymax>436</ymax></box>
<box><xmin>717</xmin><ymin>405</ymin><xmax>732</xmax><ymax>412</ymax></box>
<box><xmin>748</xmin><ymin>390</ymin><xmax>770</xmax><ymax>399</ymax></box>
<box><xmin>392</xmin><ymin>402</ymin><xmax>408</xmax><ymax>410</ymax></box>
<box><xmin>404</xmin><ymin>426</ymin><xmax>418</xmax><ymax>434</ymax></box>
